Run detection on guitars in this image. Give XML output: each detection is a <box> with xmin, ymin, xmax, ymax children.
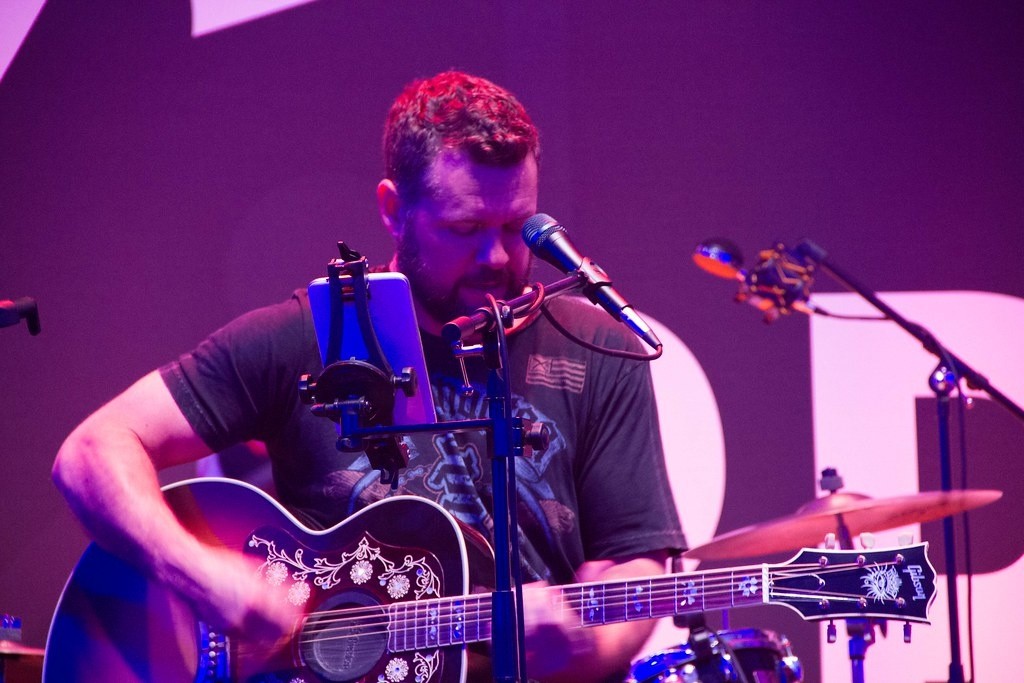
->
<box><xmin>41</xmin><ymin>470</ymin><xmax>946</xmax><ymax>682</ymax></box>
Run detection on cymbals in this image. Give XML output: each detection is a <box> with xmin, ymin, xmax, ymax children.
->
<box><xmin>680</xmin><ymin>487</ymin><xmax>1006</xmax><ymax>561</ymax></box>
<box><xmin>0</xmin><ymin>639</ymin><xmax>46</xmax><ymax>660</ymax></box>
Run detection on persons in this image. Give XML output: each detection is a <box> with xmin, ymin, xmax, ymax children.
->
<box><xmin>51</xmin><ymin>69</ymin><xmax>687</xmax><ymax>683</ymax></box>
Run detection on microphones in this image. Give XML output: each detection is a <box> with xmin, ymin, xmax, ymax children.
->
<box><xmin>519</xmin><ymin>213</ymin><xmax>662</xmax><ymax>350</ymax></box>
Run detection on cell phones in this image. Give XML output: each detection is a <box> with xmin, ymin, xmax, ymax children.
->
<box><xmin>308</xmin><ymin>273</ymin><xmax>438</xmax><ymax>425</ymax></box>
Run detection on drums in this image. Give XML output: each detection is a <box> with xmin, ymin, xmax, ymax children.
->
<box><xmin>630</xmin><ymin>623</ymin><xmax>804</xmax><ymax>682</ymax></box>
